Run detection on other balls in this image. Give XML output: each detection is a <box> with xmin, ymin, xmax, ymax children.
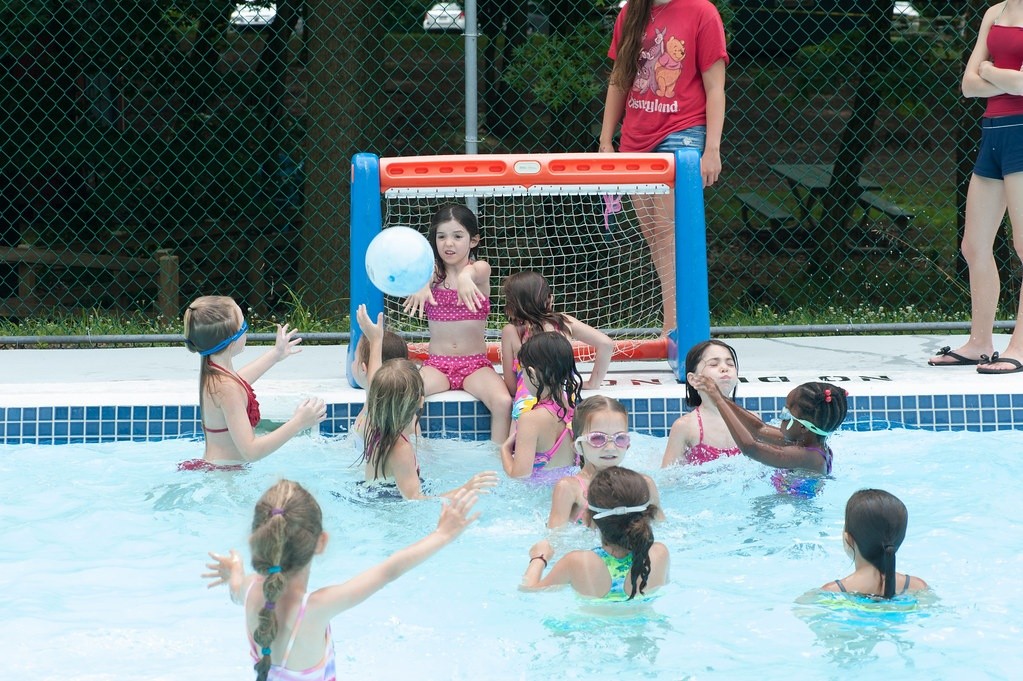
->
<box><xmin>365</xmin><ymin>224</ymin><xmax>436</xmax><ymax>299</ymax></box>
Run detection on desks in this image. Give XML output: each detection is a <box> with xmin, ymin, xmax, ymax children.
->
<box><xmin>767</xmin><ymin>162</ymin><xmax>884</xmax><ymax>255</ymax></box>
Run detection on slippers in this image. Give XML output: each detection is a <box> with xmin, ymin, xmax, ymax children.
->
<box><xmin>927</xmin><ymin>343</ymin><xmax>994</xmax><ymax>376</ymax></box>
<box><xmin>976</xmin><ymin>350</ymin><xmax>1023</xmax><ymax>375</ymax></box>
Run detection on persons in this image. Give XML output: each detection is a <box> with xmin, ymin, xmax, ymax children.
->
<box><xmin>598</xmin><ymin>0</ymin><xmax>729</xmax><ymax>337</ymax></box>
<box><xmin>661</xmin><ymin>339</ymin><xmax>762</xmax><ymax>470</ymax></box>
<box><xmin>820</xmin><ymin>488</ymin><xmax>928</xmax><ymax>597</ymax></box>
<box><xmin>182</xmin><ymin>296</ymin><xmax>327</xmax><ymax>465</ymax></box>
<box><xmin>693</xmin><ymin>374</ymin><xmax>848</xmax><ymax>475</ymax></box>
<box><xmin>200</xmin><ymin>478</ymin><xmax>483</xmax><ymax>681</ymax></box>
<box><xmin>352</xmin><ymin>303</ymin><xmax>500</xmax><ymax>501</ymax></box>
<box><xmin>502</xmin><ymin>271</ymin><xmax>613</xmax><ymax>435</ymax></box>
<box><xmin>517</xmin><ymin>466</ymin><xmax>670</xmax><ymax>598</ymax></box>
<box><xmin>927</xmin><ymin>0</ymin><xmax>1023</xmax><ymax>373</ymax></box>
<box><xmin>500</xmin><ymin>332</ymin><xmax>583</xmax><ymax>478</ymax></box>
<box><xmin>546</xmin><ymin>394</ymin><xmax>666</xmax><ymax>529</ymax></box>
<box><xmin>403</xmin><ymin>203</ymin><xmax>511</xmax><ymax>445</ymax></box>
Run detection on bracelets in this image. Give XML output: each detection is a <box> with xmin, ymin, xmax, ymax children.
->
<box><xmin>530</xmin><ymin>555</ymin><xmax>547</xmax><ymax>567</ymax></box>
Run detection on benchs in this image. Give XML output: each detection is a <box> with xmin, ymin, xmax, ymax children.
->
<box><xmin>858</xmin><ymin>191</ymin><xmax>919</xmax><ymax>255</ymax></box>
<box><xmin>730</xmin><ymin>191</ymin><xmax>798</xmax><ymax>248</ymax></box>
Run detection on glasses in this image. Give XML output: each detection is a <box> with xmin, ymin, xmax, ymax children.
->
<box><xmin>779</xmin><ymin>406</ymin><xmax>833</xmax><ymax>439</ymax></box>
<box><xmin>575</xmin><ymin>433</ymin><xmax>633</xmax><ymax>451</ymax></box>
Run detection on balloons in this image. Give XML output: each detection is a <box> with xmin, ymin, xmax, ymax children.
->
<box><xmin>365</xmin><ymin>226</ymin><xmax>435</xmax><ymax>297</ymax></box>
